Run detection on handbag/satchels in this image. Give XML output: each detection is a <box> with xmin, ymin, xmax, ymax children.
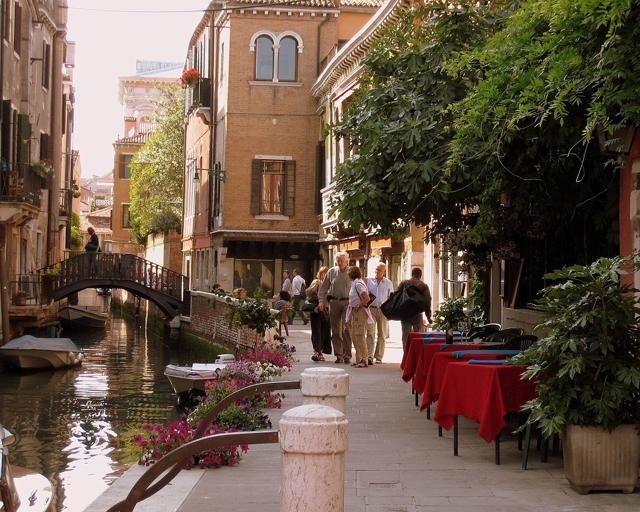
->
<box><xmin>301</xmin><ymin>294</ymin><xmax>319</xmax><ymax>313</ymax></box>
<box><xmin>360</xmin><ymin>292</ymin><xmax>377</xmax><ymax>308</ymax></box>
<box><xmin>85</xmin><ymin>242</ymin><xmax>99</xmax><ymax>251</ymax></box>
<box><xmin>378</xmin><ymin>284</ymin><xmax>427</xmax><ymax>322</ymax></box>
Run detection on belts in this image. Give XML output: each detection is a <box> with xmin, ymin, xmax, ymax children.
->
<box><xmin>330</xmin><ymin>296</ymin><xmax>349</xmax><ymax>301</ymax></box>
<box><xmin>370</xmin><ymin>305</ymin><xmax>381</xmax><ymax>309</ymax></box>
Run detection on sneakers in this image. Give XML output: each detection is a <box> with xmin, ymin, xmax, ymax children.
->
<box><xmin>311</xmin><ymin>354</ymin><xmax>319</xmax><ymax>361</ymax></box>
<box><xmin>319</xmin><ymin>354</ymin><xmax>325</xmax><ymax>361</ymax></box>
<box><xmin>355</xmin><ymin>361</ymin><xmax>369</xmax><ymax>368</ymax></box>
<box><xmin>351</xmin><ymin>362</ymin><xmax>357</xmax><ymax>366</ymax></box>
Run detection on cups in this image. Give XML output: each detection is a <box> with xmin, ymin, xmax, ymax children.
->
<box><xmin>473</xmin><ymin>339</ymin><xmax>482</xmax><ymax>344</ymax></box>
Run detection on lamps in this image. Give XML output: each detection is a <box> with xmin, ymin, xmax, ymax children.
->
<box><xmin>192</xmin><ymin>166</ymin><xmax>225</xmax><ymax>183</ymax></box>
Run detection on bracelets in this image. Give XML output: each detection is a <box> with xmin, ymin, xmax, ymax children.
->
<box><xmin>319</xmin><ymin>299</ymin><xmax>324</xmax><ymax>302</ymax></box>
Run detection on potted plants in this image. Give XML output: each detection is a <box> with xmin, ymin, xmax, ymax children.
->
<box><xmin>506</xmin><ymin>250</ymin><xmax>640</xmax><ymax>495</ymax></box>
<box><xmin>43</xmin><ymin>270</ymin><xmax>60</xmax><ymax>280</ymax></box>
<box><xmin>11</xmin><ymin>290</ymin><xmax>29</xmax><ymax>305</ymax></box>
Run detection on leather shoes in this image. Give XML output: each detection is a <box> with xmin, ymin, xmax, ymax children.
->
<box><xmin>287</xmin><ymin>321</ymin><xmax>293</xmax><ymax>325</ymax></box>
<box><xmin>335</xmin><ymin>357</ymin><xmax>344</xmax><ymax>363</ymax></box>
<box><xmin>344</xmin><ymin>357</ymin><xmax>351</xmax><ymax>363</ymax></box>
<box><xmin>368</xmin><ymin>357</ymin><xmax>373</xmax><ymax>365</ymax></box>
<box><xmin>303</xmin><ymin>318</ymin><xmax>310</xmax><ymax>325</ymax></box>
<box><xmin>375</xmin><ymin>359</ymin><xmax>382</xmax><ymax>364</ymax></box>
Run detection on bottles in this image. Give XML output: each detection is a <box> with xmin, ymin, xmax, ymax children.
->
<box><xmin>421</xmin><ymin>325</ymin><xmax>433</xmax><ymax>332</ymax></box>
<box><xmin>446</xmin><ymin>311</ymin><xmax>454</xmax><ymax>345</ymax></box>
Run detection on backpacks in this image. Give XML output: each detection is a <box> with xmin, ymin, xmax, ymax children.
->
<box><xmin>293</xmin><ymin>276</ymin><xmax>307</xmax><ymax>300</ymax></box>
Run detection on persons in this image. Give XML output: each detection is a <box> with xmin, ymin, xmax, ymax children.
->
<box><xmin>317</xmin><ymin>252</ymin><xmax>352</xmax><ymax>364</ymax></box>
<box><xmin>305</xmin><ymin>266</ymin><xmax>333</xmax><ymax>362</ymax></box>
<box><xmin>288</xmin><ymin>268</ymin><xmax>309</xmax><ymax>325</ymax></box>
<box><xmin>211</xmin><ymin>283</ymin><xmax>291</xmax><ymax>320</ymax></box>
<box><xmin>398</xmin><ymin>268</ymin><xmax>433</xmax><ymax>350</ymax></box>
<box><xmin>280</xmin><ymin>269</ymin><xmax>292</xmax><ymax>324</ymax></box>
<box><xmin>364</xmin><ymin>262</ymin><xmax>394</xmax><ymax>365</ymax></box>
<box><xmin>345</xmin><ymin>265</ymin><xmax>375</xmax><ymax>369</ymax></box>
<box><xmin>83</xmin><ymin>227</ymin><xmax>101</xmax><ymax>277</ymax></box>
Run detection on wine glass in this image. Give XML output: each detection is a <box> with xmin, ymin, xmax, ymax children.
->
<box><xmin>464</xmin><ymin>323</ymin><xmax>471</xmax><ymax>345</ymax></box>
<box><xmin>458</xmin><ymin>321</ymin><xmax>465</xmax><ymax>344</ymax></box>
